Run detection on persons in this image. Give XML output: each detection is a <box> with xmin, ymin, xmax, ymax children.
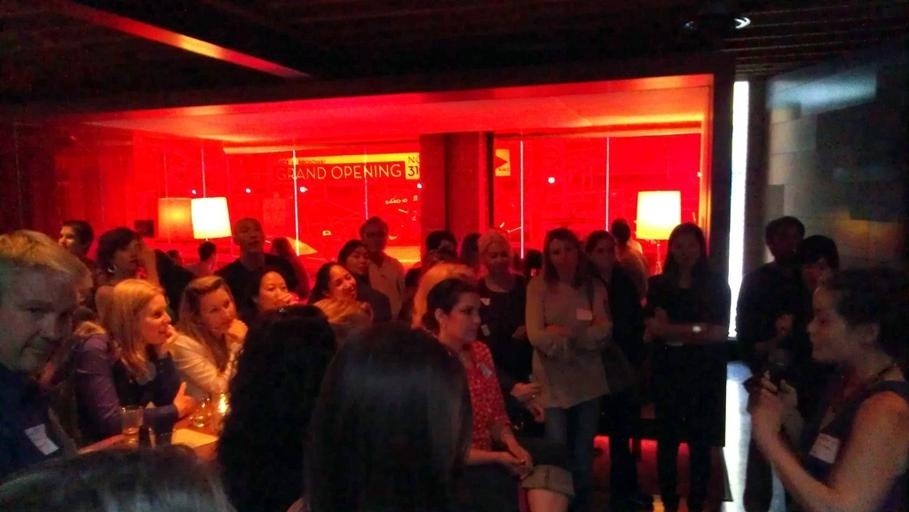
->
<box><xmin>736</xmin><ymin>215</ymin><xmax>909</xmax><ymax>511</ymax></box>
<box><xmin>1</xmin><ymin>214</ymin><xmax>731</xmax><ymax>511</ymax></box>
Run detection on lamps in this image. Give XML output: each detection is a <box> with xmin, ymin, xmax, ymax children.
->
<box><xmin>190</xmin><ymin>197</ymin><xmax>232</xmax><ymax>261</ymax></box>
<box><xmin>634</xmin><ymin>189</ymin><xmax>683</xmax><ymax>274</ymax></box>
<box><xmin>157</xmin><ymin>196</ymin><xmax>191</xmax><ymax>250</ymax></box>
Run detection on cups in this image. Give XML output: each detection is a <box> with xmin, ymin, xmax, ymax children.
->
<box><xmin>120</xmin><ymin>405</ymin><xmax>144</xmax><ymax>444</ymax></box>
<box><xmin>187</xmin><ymin>395</ymin><xmax>210</xmax><ymax>428</ymax></box>
<box><xmin>210</xmin><ymin>392</ymin><xmax>230</xmax><ymax>434</ymax></box>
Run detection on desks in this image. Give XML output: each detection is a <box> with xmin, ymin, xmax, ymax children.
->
<box><xmin>56</xmin><ymin>390</ymin><xmax>228</xmax><ymax>468</ymax></box>
